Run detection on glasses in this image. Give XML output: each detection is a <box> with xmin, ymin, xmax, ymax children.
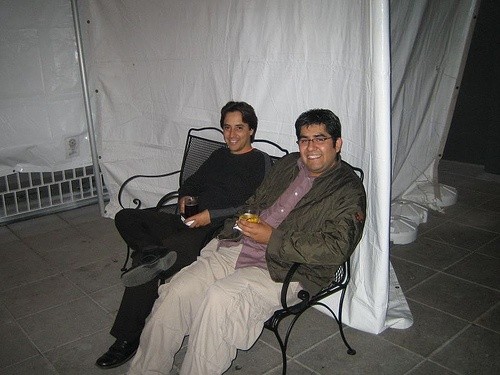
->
<box><xmin>296</xmin><ymin>136</ymin><xmax>333</xmax><ymax>145</ymax></box>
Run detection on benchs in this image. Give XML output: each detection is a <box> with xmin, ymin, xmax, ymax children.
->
<box><xmin>118</xmin><ymin>126</ymin><xmax>366</xmax><ymax>375</ymax></box>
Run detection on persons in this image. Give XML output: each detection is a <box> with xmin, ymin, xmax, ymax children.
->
<box><xmin>126</xmin><ymin>108</ymin><xmax>367</xmax><ymax>375</ymax></box>
<box><xmin>94</xmin><ymin>100</ymin><xmax>276</xmax><ymax>369</ymax></box>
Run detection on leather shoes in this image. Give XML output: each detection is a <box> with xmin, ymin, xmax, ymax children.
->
<box><xmin>96</xmin><ymin>339</ymin><xmax>139</xmax><ymax>368</ymax></box>
<box><xmin>120</xmin><ymin>248</ymin><xmax>177</xmax><ymax>287</ymax></box>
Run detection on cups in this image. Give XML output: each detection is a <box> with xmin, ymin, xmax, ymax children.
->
<box><xmin>184</xmin><ymin>196</ymin><xmax>199</xmax><ymax>219</ymax></box>
<box><xmin>238</xmin><ymin>205</ymin><xmax>259</xmax><ymax>223</ymax></box>
